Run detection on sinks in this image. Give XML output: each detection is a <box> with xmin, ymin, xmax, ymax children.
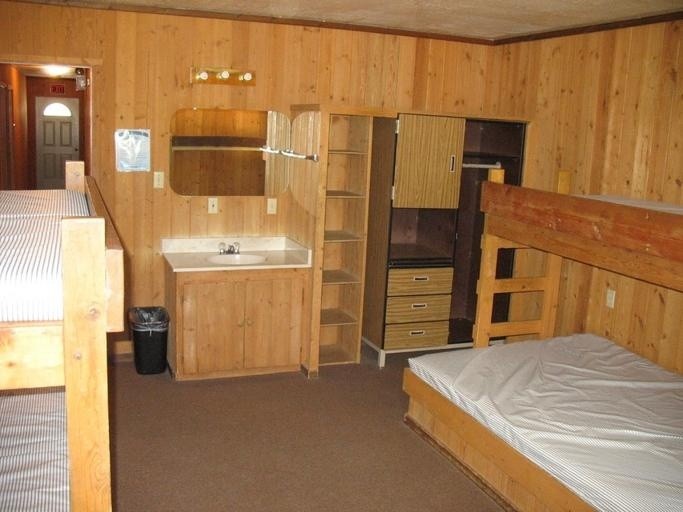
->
<box><xmin>208</xmin><ymin>253</ymin><xmax>264</xmax><ymax>265</ymax></box>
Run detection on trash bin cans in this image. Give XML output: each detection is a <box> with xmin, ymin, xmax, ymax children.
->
<box><xmin>128</xmin><ymin>306</ymin><xmax>170</xmax><ymax>375</ymax></box>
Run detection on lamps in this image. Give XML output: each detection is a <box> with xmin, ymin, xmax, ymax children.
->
<box><xmin>42</xmin><ymin>102</ymin><xmax>73</xmax><ymax>117</ymax></box>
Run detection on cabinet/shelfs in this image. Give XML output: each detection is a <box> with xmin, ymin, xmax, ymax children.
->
<box><xmin>163</xmin><ymin>260</ymin><xmax>306</xmax><ymax>383</ymax></box>
<box><xmin>362</xmin><ymin>114</ymin><xmax>528</xmax><ymax>370</ymax></box>
<box><xmin>318</xmin><ymin>113</ymin><xmax>375</xmax><ymax>367</ymax></box>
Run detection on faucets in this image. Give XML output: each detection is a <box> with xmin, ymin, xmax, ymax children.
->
<box><xmin>227</xmin><ymin>245</ymin><xmax>235</xmax><ymax>255</ymax></box>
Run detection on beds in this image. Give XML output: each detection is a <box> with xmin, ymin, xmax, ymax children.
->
<box><xmin>408</xmin><ymin>170</ymin><xmax>683</xmax><ymax>512</ymax></box>
<box><xmin>0</xmin><ymin>157</ymin><xmax>129</xmax><ymax>512</ymax></box>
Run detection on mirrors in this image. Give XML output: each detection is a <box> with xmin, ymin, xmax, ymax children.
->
<box><xmin>166</xmin><ymin>105</ymin><xmax>293</xmax><ymax>199</ymax></box>
